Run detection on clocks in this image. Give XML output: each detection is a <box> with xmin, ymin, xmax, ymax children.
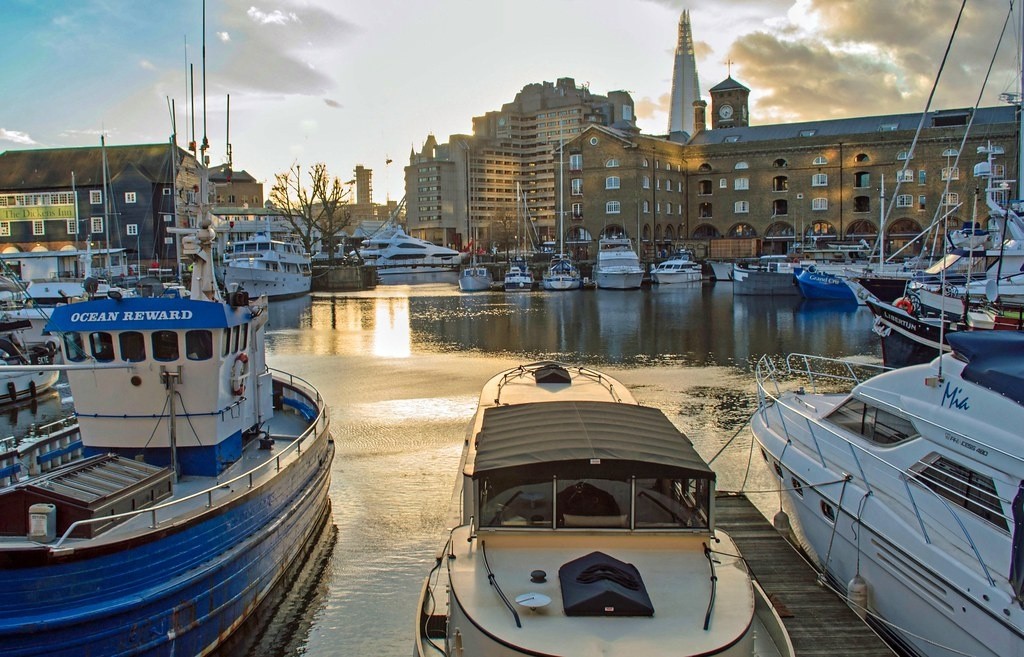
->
<box><xmin>742</xmin><ymin>104</ymin><xmax>747</xmax><ymax>118</ymax></box>
<box><xmin>719</xmin><ymin>104</ymin><xmax>733</xmax><ymax>118</ymax></box>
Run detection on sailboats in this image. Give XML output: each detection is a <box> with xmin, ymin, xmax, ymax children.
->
<box><xmin>543</xmin><ymin>119</ymin><xmax>582</xmax><ymax>289</ymax></box>
<box><xmin>71</xmin><ymin>0</ymin><xmax>232</xmax><ymax>304</ymax></box>
<box><xmin>457</xmin><ymin>174</ymin><xmax>495</xmax><ymax>292</ymax></box>
<box><xmin>504</xmin><ymin>182</ymin><xmax>535</xmax><ymax>292</ymax></box>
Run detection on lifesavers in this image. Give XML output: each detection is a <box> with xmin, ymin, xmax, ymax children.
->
<box><xmin>531</xmin><ymin>272</ymin><xmax>535</xmax><ymax>281</ymax></box>
<box><xmin>231</xmin><ymin>352</ymin><xmax>250</xmax><ymax>396</ymax></box>
<box><xmin>892</xmin><ymin>297</ymin><xmax>912</xmax><ymax>315</ymax></box>
<box><xmin>793</xmin><ymin>258</ymin><xmax>799</xmax><ymax>263</ymax></box>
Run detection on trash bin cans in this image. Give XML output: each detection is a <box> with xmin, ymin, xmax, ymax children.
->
<box><xmin>26</xmin><ymin>502</ymin><xmax>58</xmax><ymax>543</ymax></box>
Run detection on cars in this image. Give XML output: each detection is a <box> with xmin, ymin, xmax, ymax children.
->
<box><xmin>591</xmin><ymin>236</ymin><xmax>645</xmax><ymax>289</ymax></box>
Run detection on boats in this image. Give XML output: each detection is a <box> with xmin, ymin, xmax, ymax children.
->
<box><xmin>0</xmin><ymin>249</ymin><xmax>336</xmax><ymax>657</ymax></box>
<box><xmin>705</xmin><ymin>260</ymin><xmax>734</xmax><ymax>282</ymax></box>
<box><xmin>745</xmin><ymin>1</ymin><xmax>1024</xmax><ymax>656</ymax></box>
<box><xmin>413</xmin><ymin>359</ymin><xmax>797</xmax><ymax>657</ymax></box>
<box><xmin>732</xmin><ymin>246</ymin><xmax>798</xmax><ymax>295</ymax></box>
<box><xmin>216</xmin><ymin>214</ymin><xmax>313</xmax><ymax>302</ymax></box>
<box><xmin>348</xmin><ymin>223</ymin><xmax>468</xmax><ymax>274</ymax></box>
<box><xmin>650</xmin><ymin>260</ymin><xmax>703</xmax><ymax>284</ymax></box>
